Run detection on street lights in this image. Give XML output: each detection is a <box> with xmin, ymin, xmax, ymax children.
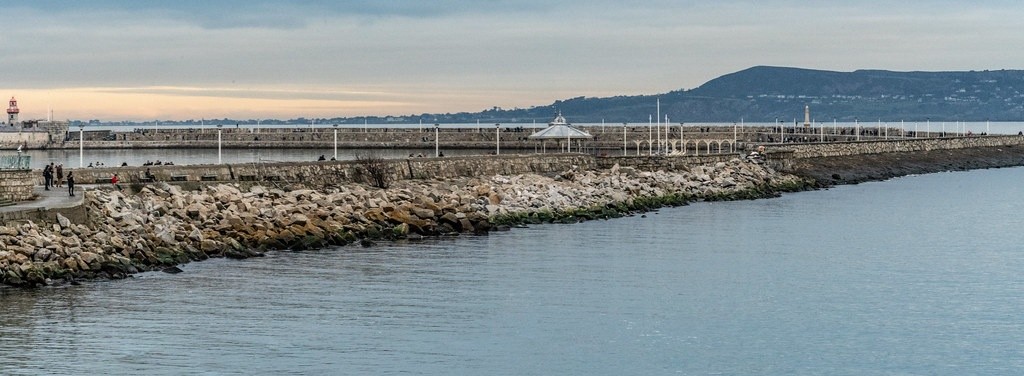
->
<box><xmin>364</xmin><ymin>117</ymin><xmax>367</xmax><ymax>133</ymax></box>
<box><xmin>650</xmin><ymin>98</ymin><xmax>668</xmax><ymax>153</ymax></box>
<box><xmin>821</xmin><ymin>122</ymin><xmax>823</xmax><ymax>142</ymax></box>
<box><xmin>734</xmin><ymin>123</ymin><xmax>737</xmax><ymax>152</ymax></box>
<box><xmin>885</xmin><ymin>124</ymin><xmax>888</xmax><ymax>139</ymax></box>
<box><xmin>956</xmin><ymin>120</ymin><xmax>958</xmax><ymax>137</ymax></box>
<box><xmin>217</xmin><ymin>123</ymin><xmax>222</xmax><ymax>164</ymax></box>
<box><xmin>79</xmin><ymin>123</ymin><xmax>85</xmax><ymax>167</ymax></box>
<box><xmin>201</xmin><ymin>118</ymin><xmax>204</xmax><ymax>133</ymax></box>
<box><xmin>901</xmin><ymin>119</ymin><xmax>904</xmax><ymax>138</ymax></box>
<box><xmin>780</xmin><ymin>121</ymin><xmax>784</xmax><ymax>143</ymax></box>
<box><xmin>741</xmin><ymin>118</ymin><xmax>743</xmax><ymax>132</ymax></box>
<box><xmin>419</xmin><ymin>117</ymin><xmax>421</xmax><ymax>133</ymax></box>
<box><xmin>927</xmin><ymin>117</ymin><xmax>930</xmax><ymax>138</ymax></box>
<box><xmin>854</xmin><ymin>116</ymin><xmax>860</xmax><ymax>141</ymax></box>
<box><xmin>156</xmin><ymin>120</ymin><xmax>158</xmax><ymax>133</ymax></box>
<box><xmin>834</xmin><ymin>116</ymin><xmax>837</xmax><ymax>135</ymax></box>
<box><xmin>601</xmin><ymin>119</ymin><xmax>604</xmax><ymax>132</ymax></box>
<box><xmin>567</xmin><ymin>124</ymin><xmax>571</xmax><ymax>152</ymax></box>
<box><xmin>914</xmin><ymin>122</ymin><xmax>918</xmax><ymax>138</ymax></box>
<box><xmin>332</xmin><ymin>123</ymin><xmax>338</xmax><ymax>160</ymax></box>
<box><xmin>775</xmin><ymin>116</ymin><xmax>778</xmax><ymax>133</ymax></box>
<box><xmin>987</xmin><ymin>117</ymin><xmax>990</xmax><ymax>135</ymax></box>
<box><xmin>257</xmin><ymin>119</ymin><xmax>259</xmax><ymax>133</ymax></box>
<box><xmin>680</xmin><ymin>122</ymin><xmax>684</xmax><ymax>152</ymax></box>
<box><xmin>813</xmin><ymin>118</ymin><xmax>815</xmax><ymax>133</ymax></box>
<box><xmin>435</xmin><ymin>123</ymin><xmax>439</xmax><ymax>157</ymax></box>
<box><xmin>963</xmin><ymin>120</ymin><xmax>965</xmax><ymax>137</ymax></box>
<box><xmin>495</xmin><ymin>123</ymin><xmax>500</xmax><ymax>155</ymax></box>
<box><xmin>532</xmin><ymin>119</ymin><xmax>535</xmax><ymax>133</ymax></box>
<box><xmin>942</xmin><ymin>120</ymin><xmax>944</xmax><ymax>138</ymax></box>
<box><xmin>793</xmin><ymin>118</ymin><xmax>796</xmax><ymax>134</ymax></box>
<box><xmin>879</xmin><ymin>117</ymin><xmax>881</xmax><ymax>137</ymax></box>
<box><xmin>623</xmin><ymin>122</ymin><xmax>627</xmax><ymax>155</ymax></box>
<box><xmin>477</xmin><ymin>118</ymin><xmax>479</xmax><ymax>132</ymax></box>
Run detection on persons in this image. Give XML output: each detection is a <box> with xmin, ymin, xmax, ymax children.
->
<box><xmin>142</xmin><ymin>159</ymin><xmax>174</xmax><ymax>166</ymax></box>
<box><xmin>88</xmin><ymin>163</ymin><xmax>93</xmax><ymax>167</ymax></box>
<box><xmin>53</xmin><ymin>165</ymin><xmax>59</xmax><ymax>185</ymax></box>
<box><xmin>317</xmin><ymin>155</ymin><xmax>335</xmax><ymax>161</ymax></box>
<box><xmin>100</xmin><ymin>162</ymin><xmax>106</xmax><ymax>168</ymax></box>
<box><xmin>146</xmin><ymin>168</ymin><xmax>156</xmax><ymax>182</ymax></box>
<box><xmin>56</xmin><ymin>164</ymin><xmax>64</xmax><ymax>187</ymax></box>
<box><xmin>111</xmin><ymin>174</ymin><xmax>124</xmax><ymax>192</ymax></box>
<box><xmin>121</xmin><ymin>162</ymin><xmax>128</xmax><ymax>166</ymax></box>
<box><xmin>67</xmin><ymin>171</ymin><xmax>75</xmax><ymax>196</ymax></box>
<box><xmin>409</xmin><ymin>150</ymin><xmax>497</xmax><ymax>159</ymax></box>
<box><xmin>95</xmin><ymin>162</ymin><xmax>100</xmax><ymax>167</ymax></box>
<box><xmin>58</xmin><ymin>163</ymin><xmax>65</xmax><ymax>187</ymax></box>
<box><xmin>43</xmin><ymin>165</ymin><xmax>50</xmax><ymax>191</ymax></box>
<box><xmin>48</xmin><ymin>162</ymin><xmax>55</xmax><ymax>187</ymax></box>
